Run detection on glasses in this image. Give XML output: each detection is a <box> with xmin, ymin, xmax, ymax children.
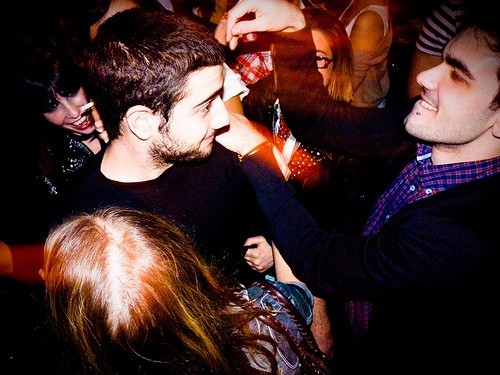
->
<box><xmin>315</xmin><ymin>56</ymin><xmax>336</xmax><ymax>69</ymax></box>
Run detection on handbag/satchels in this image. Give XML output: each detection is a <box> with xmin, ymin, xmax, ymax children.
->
<box><xmin>226</xmin><ymin>273</ymin><xmax>331</xmax><ymax>375</ymax></box>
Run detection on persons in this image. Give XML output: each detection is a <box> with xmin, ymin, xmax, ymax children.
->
<box><xmin>1</xmin><ymin>0</ymin><xmax>500</xmax><ymax>284</ymax></box>
<box><xmin>36</xmin><ymin>206</ymin><xmax>329</xmax><ymax>375</ymax></box>
<box><xmin>226</xmin><ymin>0</ymin><xmax>500</xmax><ymax>375</ymax></box>
<box><xmin>21</xmin><ymin>9</ymin><xmax>330</xmax><ymax>355</ymax></box>
